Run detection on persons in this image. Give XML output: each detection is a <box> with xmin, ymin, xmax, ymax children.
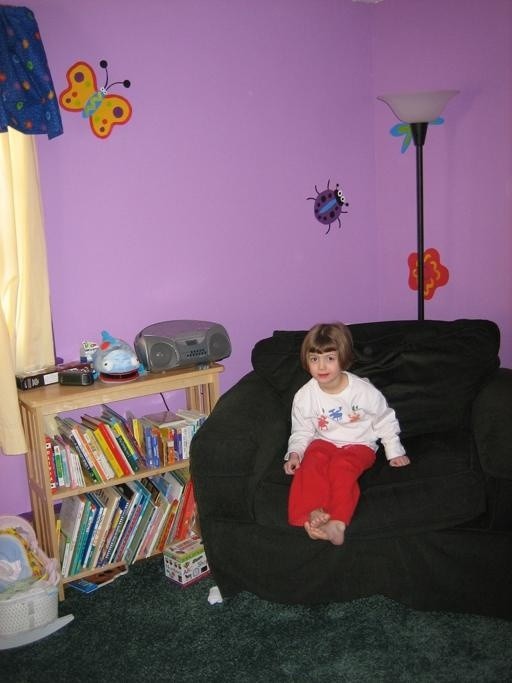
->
<box><xmin>281</xmin><ymin>321</ymin><xmax>412</xmax><ymax>547</ymax></box>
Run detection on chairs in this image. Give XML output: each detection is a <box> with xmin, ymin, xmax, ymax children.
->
<box><xmin>189</xmin><ymin>317</ymin><xmax>512</xmax><ymax>621</ymax></box>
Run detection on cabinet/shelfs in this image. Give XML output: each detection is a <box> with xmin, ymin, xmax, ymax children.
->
<box><xmin>18</xmin><ymin>362</ymin><xmax>224</xmax><ymax>602</ymax></box>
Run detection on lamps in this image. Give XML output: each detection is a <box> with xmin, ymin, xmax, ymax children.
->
<box><xmin>376</xmin><ymin>89</ymin><xmax>460</xmax><ymax>320</ymax></box>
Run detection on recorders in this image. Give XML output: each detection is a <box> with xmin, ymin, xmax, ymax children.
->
<box><xmin>135</xmin><ymin>320</ymin><xmax>232</xmax><ymax>374</ymax></box>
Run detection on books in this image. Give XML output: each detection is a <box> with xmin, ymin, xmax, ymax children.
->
<box><xmin>43</xmin><ymin>402</ymin><xmax>212</xmax><ymax>595</ymax></box>
